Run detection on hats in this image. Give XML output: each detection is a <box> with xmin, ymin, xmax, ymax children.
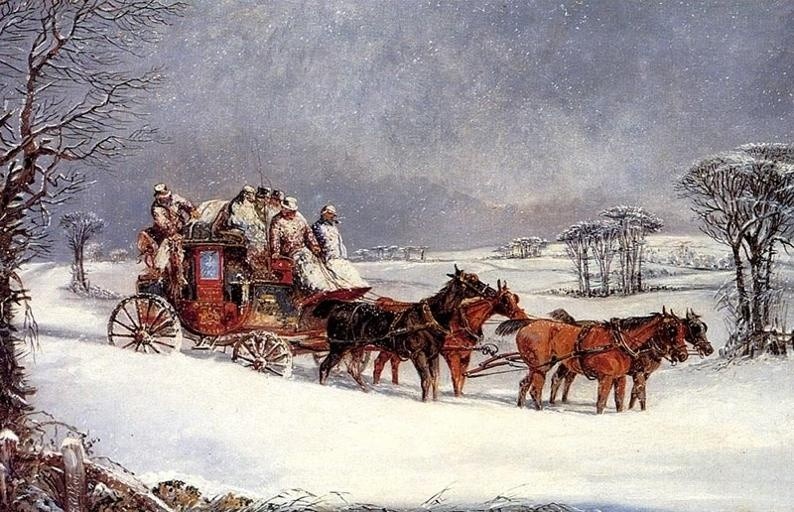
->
<box><xmin>242</xmin><ymin>186</ymin><xmax>256</xmax><ymax>200</ymax></box>
<box><xmin>154</xmin><ymin>184</ymin><xmax>171</xmax><ymax>199</ymax></box>
<box><xmin>269</xmin><ymin>189</ymin><xmax>285</xmax><ymax>201</ymax></box>
<box><xmin>320</xmin><ymin>204</ymin><xmax>337</xmax><ymax>217</ymax></box>
<box><xmin>281</xmin><ymin>197</ymin><xmax>298</xmax><ymax>211</ymax></box>
<box><xmin>255</xmin><ymin>186</ymin><xmax>271</xmax><ymax>199</ymax></box>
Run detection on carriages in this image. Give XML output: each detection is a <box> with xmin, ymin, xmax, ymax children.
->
<box><xmin>104</xmin><ymin>225</ymin><xmax>715</xmax><ymax>414</ymax></box>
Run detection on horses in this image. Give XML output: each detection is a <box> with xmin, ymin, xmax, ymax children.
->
<box><xmin>307</xmin><ymin>263</ymin><xmax>498</xmax><ymax>402</ymax></box>
<box><xmin>495</xmin><ymin>309</ymin><xmax>691</xmax><ymax>416</ymax></box>
<box><xmin>371</xmin><ymin>279</ymin><xmax>529</xmax><ymax>397</ymax></box>
<box><xmin>547</xmin><ymin>306</ymin><xmax>713</xmax><ymax>412</ymax></box>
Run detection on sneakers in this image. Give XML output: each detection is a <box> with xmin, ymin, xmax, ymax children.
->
<box><xmin>139</xmin><ymin>283</ymin><xmax>162</xmax><ymax>295</ymax></box>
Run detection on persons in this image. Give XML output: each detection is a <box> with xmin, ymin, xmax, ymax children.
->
<box><xmin>150</xmin><ymin>181</ymin><xmax>348</xmax><ymax>310</ymax></box>
